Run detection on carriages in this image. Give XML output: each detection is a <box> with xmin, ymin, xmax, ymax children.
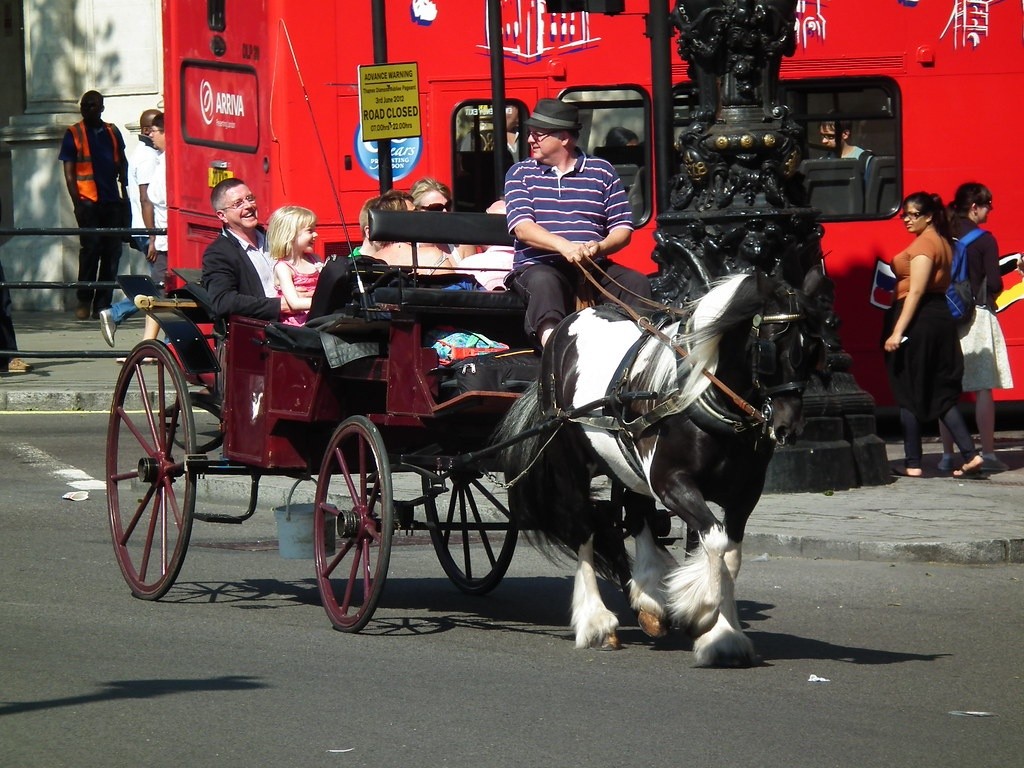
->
<box><xmin>105</xmin><ymin>204</ymin><xmax>812</xmax><ymax>668</ymax></box>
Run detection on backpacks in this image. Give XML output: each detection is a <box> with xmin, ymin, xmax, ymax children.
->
<box><xmin>943</xmin><ymin>228</ymin><xmax>985</xmax><ymax>323</ymax></box>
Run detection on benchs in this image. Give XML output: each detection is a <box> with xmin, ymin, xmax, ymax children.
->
<box><xmin>365</xmin><ymin>207</ymin><xmax>577</xmax><ymax>397</ymax></box>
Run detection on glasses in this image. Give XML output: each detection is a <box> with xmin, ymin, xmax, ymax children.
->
<box><xmin>900</xmin><ymin>212</ymin><xmax>922</xmax><ymax>220</ymax></box>
<box><xmin>528</xmin><ymin>130</ymin><xmax>554</xmax><ymax>141</ymax></box>
<box><xmin>982</xmin><ymin>201</ymin><xmax>992</xmax><ymax>209</ymax></box>
<box><xmin>422</xmin><ymin>200</ymin><xmax>452</xmax><ymax>212</ymax></box>
<box><xmin>819</xmin><ymin>132</ymin><xmax>837</xmax><ymax>140</ymax></box>
<box><xmin>224</xmin><ymin>194</ymin><xmax>254</xmax><ymax>211</ymax></box>
<box><xmin>150</xmin><ymin>128</ymin><xmax>163</xmax><ymax>134</ymax></box>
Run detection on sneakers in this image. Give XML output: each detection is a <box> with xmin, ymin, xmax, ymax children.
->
<box><xmin>980</xmin><ymin>457</ymin><xmax>1010</xmax><ymax>471</ymax></box>
<box><xmin>937</xmin><ymin>458</ymin><xmax>954</xmax><ymax>470</ymax></box>
<box><xmin>100</xmin><ymin>310</ymin><xmax>116</xmax><ymax>348</ymax></box>
<box><xmin>0</xmin><ymin>357</ymin><xmax>33</xmax><ymax>372</ymax></box>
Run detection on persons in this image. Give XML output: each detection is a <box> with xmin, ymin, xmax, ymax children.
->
<box><xmin>503</xmin><ymin>97</ymin><xmax>653</xmax><ymax>347</ymax></box>
<box><xmin>58</xmin><ymin>90</ymin><xmax>129</xmax><ymax>319</ymax></box>
<box><xmin>200</xmin><ymin>178</ymin><xmax>301</xmax><ymax>322</ymax></box>
<box><xmin>267</xmin><ymin>206</ymin><xmax>323</xmax><ymax>327</ymax></box>
<box><xmin>603</xmin><ymin>127</ymin><xmax>638</xmax><ymax>146</ymax></box>
<box><xmin>938</xmin><ymin>183</ymin><xmax>1009</xmax><ymax>471</ymax></box>
<box><xmin>347</xmin><ymin>179</ymin><xmax>515</xmax><ymax>292</ymax></box>
<box><xmin>818</xmin><ymin>121</ymin><xmax>872</xmax><ymax>166</ymax></box>
<box><xmin>0</xmin><ymin>263</ymin><xmax>34</xmax><ymax>372</ymax></box>
<box><xmin>100</xmin><ymin>110</ymin><xmax>169</xmax><ymax>364</ymax></box>
<box><xmin>880</xmin><ymin>191</ymin><xmax>983</xmax><ymax>477</ymax></box>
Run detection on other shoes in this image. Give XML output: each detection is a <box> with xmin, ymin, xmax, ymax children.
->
<box><xmin>117</xmin><ymin>353</ymin><xmax>157</xmax><ymax>365</ymax></box>
<box><xmin>76</xmin><ymin>306</ymin><xmax>90</xmax><ymax>319</ymax></box>
<box><xmin>92</xmin><ymin>307</ymin><xmax>105</xmax><ymax>319</ymax></box>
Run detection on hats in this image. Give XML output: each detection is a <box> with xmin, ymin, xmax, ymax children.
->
<box><xmin>524</xmin><ymin>97</ymin><xmax>582</xmax><ymax>131</ymax></box>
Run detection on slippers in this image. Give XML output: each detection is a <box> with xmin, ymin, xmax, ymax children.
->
<box><xmin>952</xmin><ymin>466</ymin><xmax>987</xmax><ymax>479</ymax></box>
<box><xmin>892</xmin><ymin>466</ymin><xmax>923</xmax><ymax>478</ymax></box>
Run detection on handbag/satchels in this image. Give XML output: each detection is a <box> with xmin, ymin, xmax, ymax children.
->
<box><xmin>431</xmin><ymin>331</ymin><xmax>509</xmax><ymax>366</ymax></box>
<box><xmin>440</xmin><ymin>352</ymin><xmax>542</xmax><ymax>400</ymax></box>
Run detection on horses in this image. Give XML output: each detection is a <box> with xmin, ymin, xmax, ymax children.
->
<box><xmin>498</xmin><ymin>271</ymin><xmax>825</xmax><ymax>671</ymax></box>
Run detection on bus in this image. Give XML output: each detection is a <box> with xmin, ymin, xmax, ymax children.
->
<box><xmin>163</xmin><ymin>1</ymin><xmax>1023</xmax><ymax>403</ymax></box>
